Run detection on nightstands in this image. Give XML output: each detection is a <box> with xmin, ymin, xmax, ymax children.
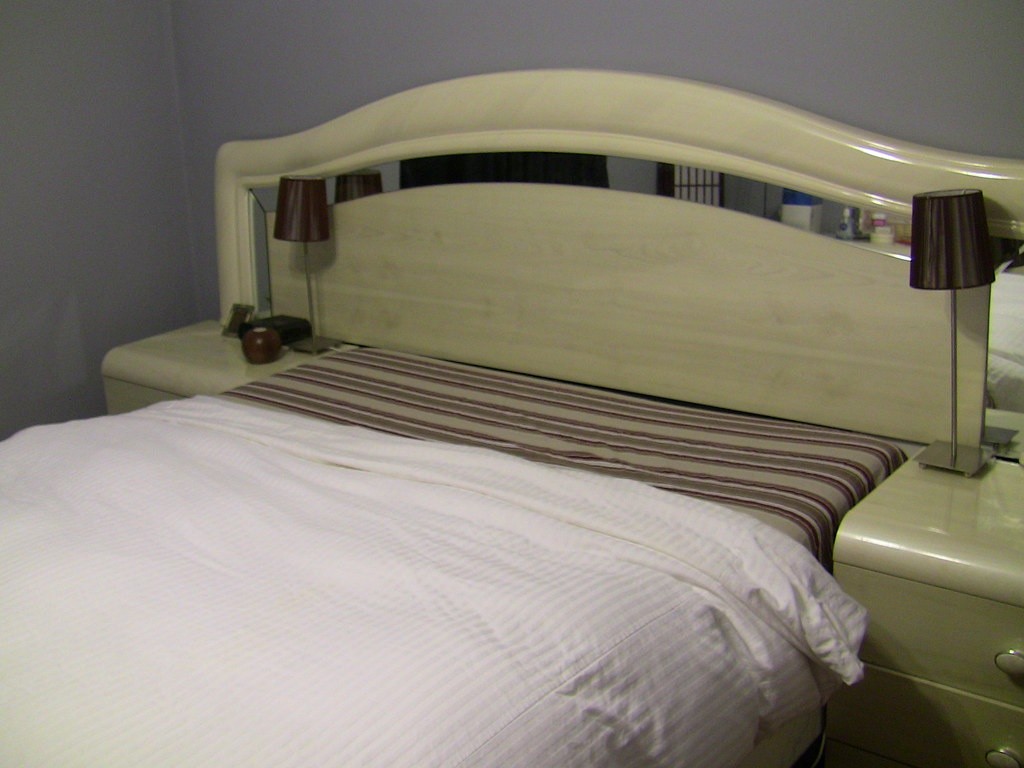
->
<box><xmin>104</xmin><ymin>318</ymin><xmax>347</xmax><ymax>419</ymax></box>
<box><xmin>825</xmin><ymin>449</ymin><xmax>1024</xmax><ymax>768</ymax></box>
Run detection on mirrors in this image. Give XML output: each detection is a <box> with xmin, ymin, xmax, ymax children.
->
<box><xmin>977</xmin><ymin>232</ymin><xmax>1024</xmax><ymax>463</ymax></box>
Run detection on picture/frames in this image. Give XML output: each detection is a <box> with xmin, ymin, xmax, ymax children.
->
<box><xmin>220</xmin><ymin>303</ymin><xmax>256</xmax><ymax>338</ymax></box>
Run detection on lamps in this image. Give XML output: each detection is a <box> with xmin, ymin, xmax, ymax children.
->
<box><xmin>985</xmin><ymin>423</ymin><xmax>1019</xmax><ymax>454</ymax></box>
<box><xmin>272</xmin><ymin>175</ymin><xmax>341</xmax><ymax>355</ymax></box>
<box><xmin>909</xmin><ymin>188</ymin><xmax>997</xmax><ymax>477</ymax></box>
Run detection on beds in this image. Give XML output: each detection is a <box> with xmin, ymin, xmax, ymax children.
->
<box><xmin>0</xmin><ymin>68</ymin><xmax>1024</xmax><ymax>768</ymax></box>
